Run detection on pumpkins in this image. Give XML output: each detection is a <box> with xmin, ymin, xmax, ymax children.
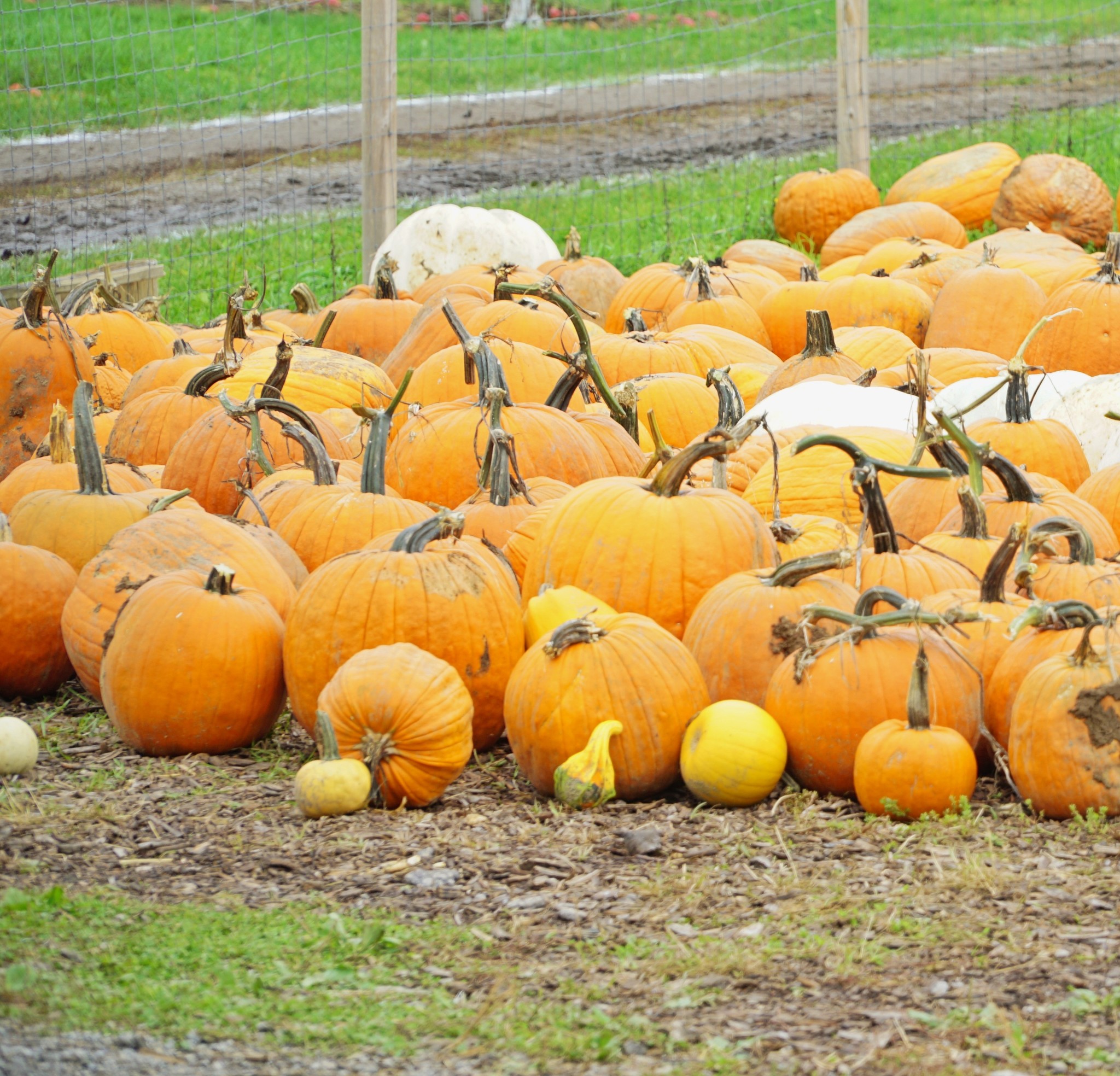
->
<box><xmin>196</xmin><ymin>0</ymin><xmax>721</xmax><ymax>31</ymax></box>
<box><xmin>0</xmin><ymin>141</ymin><xmax>1118</xmax><ymax>822</ymax></box>
